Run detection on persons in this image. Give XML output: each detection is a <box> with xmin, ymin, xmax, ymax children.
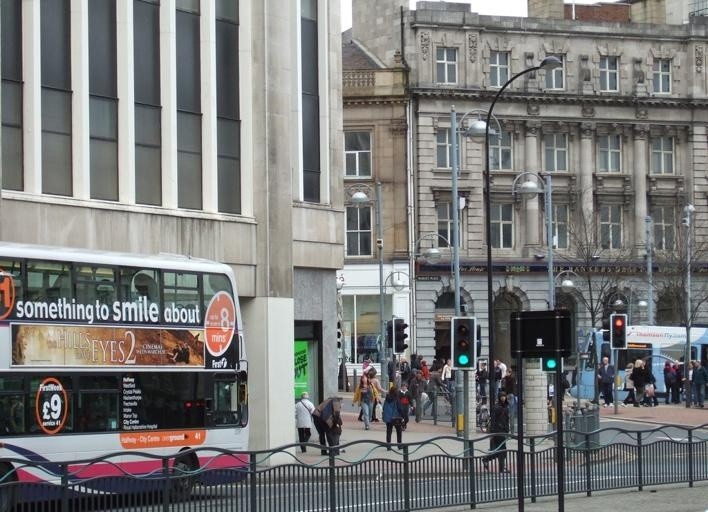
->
<box><xmin>590</xmin><ymin>357</ymin><xmax>659</xmax><ymax>409</ymax></box>
<box><xmin>664</xmin><ymin>358</ymin><xmax>708</xmax><ymax>408</ymax></box>
<box><xmin>382</xmin><ymin>387</ymin><xmax>408</xmax><ymax>450</ymax></box>
<box><xmin>482</xmin><ymin>391</ymin><xmax>511</xmax><ymax>473</ymax></box>
<box><xmin>295</xmin><ymin>392</ymin><xmax>344</xmax><ymax>456</ymax></box>
<box><xmin>193</xmin><ymin>333</ymin><xmax>200</xmax><ymax>354</ymax></box>
<box><xmin>166</xmin><ymin>341</ymin><xmax>190</xmax><ymax>364</ymax></box>
<box><xmin>475</xmin><ymin>358</ymin><xmax>514</xmax><ymax>406</ymax></box>
<box><xmin>352</xmin><ymin>354</ymin><xmax>456</xmax><ymax>430</ymax></box>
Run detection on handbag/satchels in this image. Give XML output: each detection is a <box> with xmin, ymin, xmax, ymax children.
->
<box><xmin>645</xmin><ymin>384</ymin><xmax>654</xmax><ymax>398</ymax></box>
<box><xmin>375</xmin><ymin>404</ymin><xmax>383</xmax><ymax>420</ymax></box>
<box><xmin>486</xmin><ymin>417</ymin><xmax>492</xmax><ymax>432</ymax></box>
<box><xmin>622</xmin><ymin>383</ymin><xmax>629</xmax><ymax>391</ymax></box>
<box><xmin>393</xmin><ymin>417</ymin><xmax>403</xmax><ymax>425</ymax></box>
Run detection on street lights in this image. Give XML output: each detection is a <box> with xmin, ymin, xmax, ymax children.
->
<box><xmin>681</xmin><ymin>202</ymin><xmax>696</xmax><ymax>408</ymax></box>
<box><xmin>482</xmin><ymin>53</ymin><xmax>564</xmax><ymax>418</ymax></box>
<box><xmin>340</xmin><ymin>176</ymin><xmax>411</xmax><ymax>395</ymax></box>
<box><xmin>437</xmin><ymin>99</ymin><xmax>507</xmax><ymax>438</ymax></box>
<box><xmin>510</xmin><ymin>164</ymin><xmax>583</xmax><ymax>311</ymax></box>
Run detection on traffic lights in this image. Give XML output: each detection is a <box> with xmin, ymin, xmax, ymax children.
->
<box><xmin>541</xmin><ymin>356</ymin><xmax>563</xmax><ymax>374</ymax></box>
<box><xmin>450</xmin><ymin>316</ymin><xmax>478</xmax><ymax>371</ymax></box>
<box><xmin>392</xmin><ymin>318</ymin><xmax>408</xmax><ymax>355</ymax></box>
<box><xmin>610</xmin><ymin>313</ymin><xmax>627</xmax><ymax>350</ymax></box>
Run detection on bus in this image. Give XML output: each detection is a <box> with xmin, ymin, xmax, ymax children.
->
<box><xmin>569</xmin><ymin>324</ymin><xmax>708</xmax><ymax>403</ymax></box>
<box><xmin>0</xmin><ymin>238</ymin><xmax>252</xmax><ymax>512</ymax></box>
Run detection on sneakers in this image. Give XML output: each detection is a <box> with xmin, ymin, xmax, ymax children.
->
<box><xmin>358</xmin><ymin>417</ymin><xmax>378</xmax><ymax>429</ymax></box>
<box><xmin>387</xmin><ymin>446</ymin><xmax>403</xmax><ymax>450</ymax></box>
<box><xmin>665</xmin><ymin>399</ymin><xmax>705</xmax><ymax>407</ymax></box>
<box><xmin>604</xmin><ymin>401</ymin><xmax>658</xmax><ymax>407</ymax></box>
<box><xmin>500</xmin><ymin>468</ymin><xmax>510</xmax><ymax>473</ymax></box>
<box><xmin>483</xmin><ymin>459</ymin><xmax>489</xmax><ymax>469</ymax></box>
<box><xmin>321</xmin><ymin>450</ymin><xmax>339</xmax><ymax>455</ymax></box>
